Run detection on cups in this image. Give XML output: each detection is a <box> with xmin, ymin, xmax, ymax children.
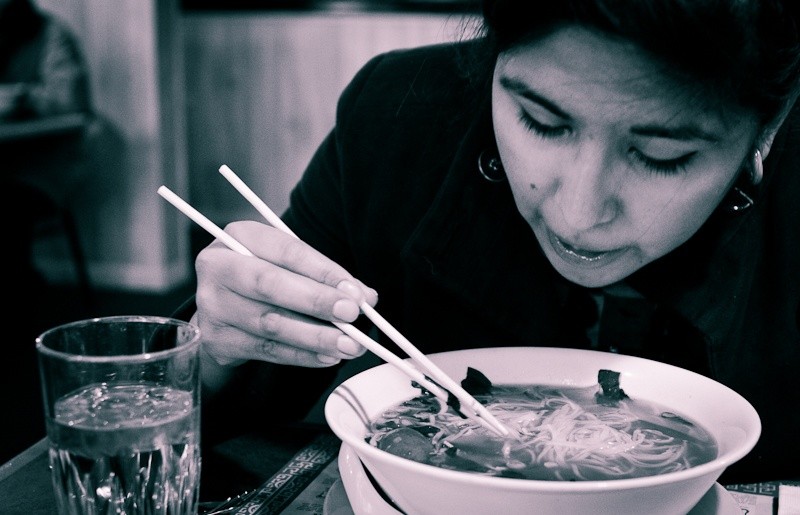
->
<box><xmin>36</xmin><ymin>315</ymin><xmax>202</xmax><ymax>515</ymax></box>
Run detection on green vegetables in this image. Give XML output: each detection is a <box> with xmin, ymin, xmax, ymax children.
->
<box><xmin>377</xmin><ymin>367</ymin><xmax>695</xmax><ymax>479</ymax></box>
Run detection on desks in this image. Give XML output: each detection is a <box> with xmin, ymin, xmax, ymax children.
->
<box><xmin>1</xmin><ymin>113</ymin><xmax>99</xmax><ymax>320</ymax></box>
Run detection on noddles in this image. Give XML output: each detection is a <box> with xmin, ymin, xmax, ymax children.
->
<box><xmin>368</xmin><ymin>389</ymin><xmax>706</xmax><ymax>481</ymax></box>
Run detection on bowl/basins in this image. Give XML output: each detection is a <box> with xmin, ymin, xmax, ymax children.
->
<box><xmin>324</xmin><ymin>346</ymin><xmax>762</xmax><ymax>515</ymax></box>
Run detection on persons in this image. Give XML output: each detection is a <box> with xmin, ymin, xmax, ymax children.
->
<box><xmin>143</xmin><ymin>0</ymin><xmax>800</xmax><ymax>420</ymax></box>
<box><xmin>0</xmin><ymin>0</ymin><xmax>92</xmax><ymax>280</ymax></box>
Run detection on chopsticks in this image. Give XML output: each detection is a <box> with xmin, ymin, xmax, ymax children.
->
<box><xmin>156</xmin><ymin>164</ymin><xmax>513</xmax><ymax>438</ymax></box>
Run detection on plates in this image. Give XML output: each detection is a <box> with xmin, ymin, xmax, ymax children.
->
<box><xmin>324</xmin><ymin>442</ymin><xmax>743</xmax><ymax>515</ymax></box>
